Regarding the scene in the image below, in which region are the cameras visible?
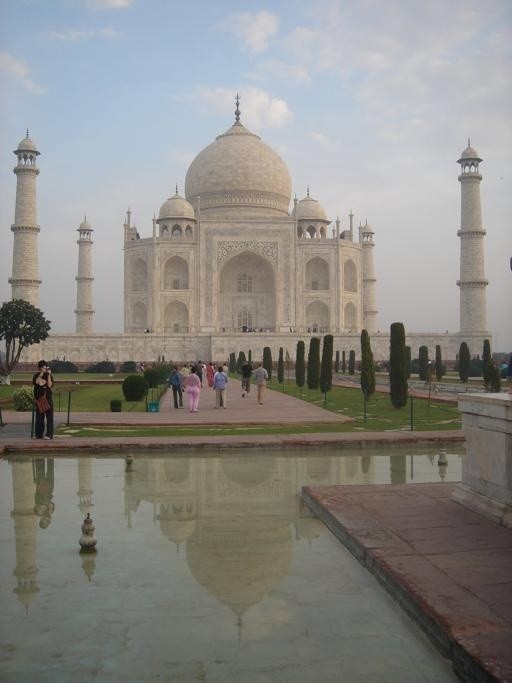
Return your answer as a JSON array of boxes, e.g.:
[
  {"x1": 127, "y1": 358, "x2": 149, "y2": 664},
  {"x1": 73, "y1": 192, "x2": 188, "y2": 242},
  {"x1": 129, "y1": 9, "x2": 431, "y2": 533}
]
[{"x1": 44, "y1": 366, "x2": 50, "y2": 372}]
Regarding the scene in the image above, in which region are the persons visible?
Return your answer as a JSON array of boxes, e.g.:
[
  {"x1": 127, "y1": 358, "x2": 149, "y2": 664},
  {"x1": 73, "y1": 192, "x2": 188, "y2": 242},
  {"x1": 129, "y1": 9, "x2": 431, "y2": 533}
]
[
  {"x1": 240, "y1": 360, "x2": 253, "y2": 397},
  {"x1": 253, "y1": 361, "x2": 269, "y2": 404},
  {"x1": 31, "y1": 360, "x2": 54, "y2": 440},
  {"x1": 501, "y1": 360, "x2": 507, "y2": 369},
  {"x1": 33, "y1": 456, "x2": 56, "y2": 529},
  {"x1": 166, "y1": 359, "x2": 229, "y2": 412}
]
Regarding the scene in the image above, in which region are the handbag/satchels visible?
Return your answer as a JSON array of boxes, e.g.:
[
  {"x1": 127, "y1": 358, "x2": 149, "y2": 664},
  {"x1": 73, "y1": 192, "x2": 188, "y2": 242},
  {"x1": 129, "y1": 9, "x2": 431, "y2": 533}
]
[{"x1": 35, "y1": 397, "x2": 51, "y2": 413}]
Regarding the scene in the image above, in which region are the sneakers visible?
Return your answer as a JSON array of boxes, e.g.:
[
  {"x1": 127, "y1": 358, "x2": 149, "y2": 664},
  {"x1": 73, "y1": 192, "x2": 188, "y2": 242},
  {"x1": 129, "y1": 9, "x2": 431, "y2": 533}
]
[
  {"x1": 43, "y1": 434, "x2": 53, "y2": 440},
  {"x1": 32, "y1": 434, "x2": 42, "y2": 439}
]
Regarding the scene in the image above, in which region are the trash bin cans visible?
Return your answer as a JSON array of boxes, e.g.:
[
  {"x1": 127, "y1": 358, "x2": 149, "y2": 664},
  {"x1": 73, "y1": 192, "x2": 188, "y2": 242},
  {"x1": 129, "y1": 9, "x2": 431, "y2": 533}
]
[
  {"x1": 110, "y1": 400, "x2": 122, "y2": 412},
  {"x1": 149, "y1": 402, "x2": 159, "y2": 412}
]
